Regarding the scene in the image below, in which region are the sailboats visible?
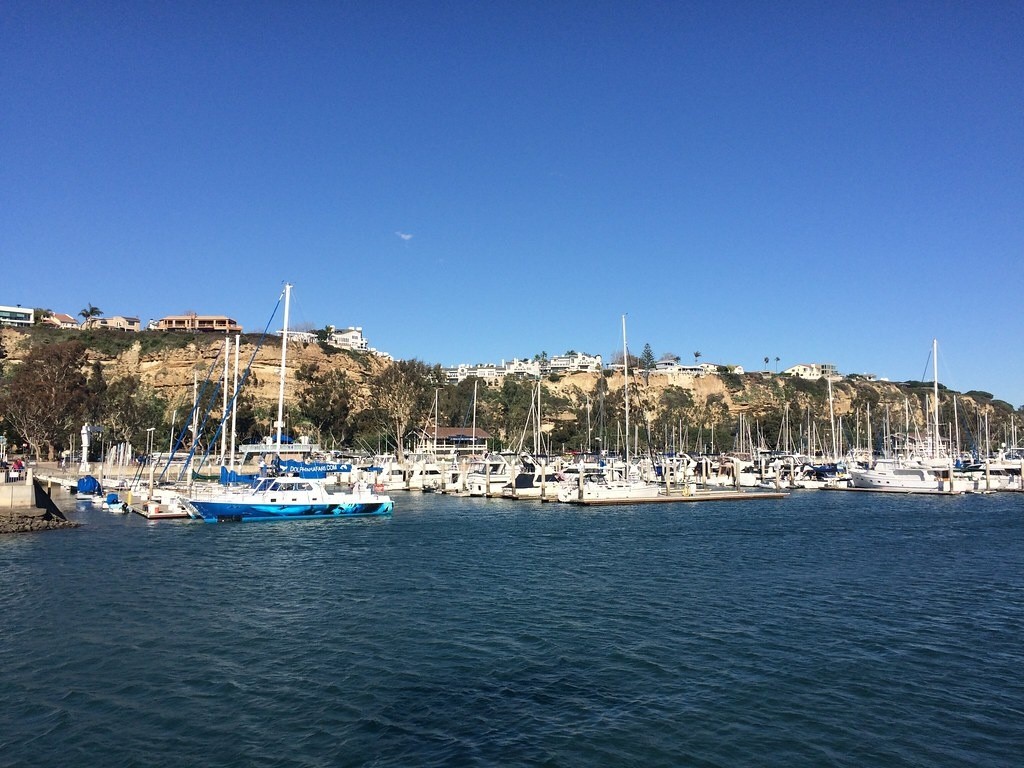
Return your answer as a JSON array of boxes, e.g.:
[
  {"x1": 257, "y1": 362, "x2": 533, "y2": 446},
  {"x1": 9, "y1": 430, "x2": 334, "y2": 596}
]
[{"x1": 77, "y1": 281, "x2": 1024, "y2": 523}]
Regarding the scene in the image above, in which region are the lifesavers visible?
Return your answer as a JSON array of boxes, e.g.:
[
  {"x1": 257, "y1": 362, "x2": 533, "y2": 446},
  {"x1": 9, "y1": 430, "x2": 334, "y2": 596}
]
[
  {"x1": 112, "y1": 499, "x2": 118, "y2": 504},
  {"x1": 122, "y1": 502, "x2": 128, "y2": 508},
  {"x1": 374, "y1": 483, "x2": 384, "y2": 492}
]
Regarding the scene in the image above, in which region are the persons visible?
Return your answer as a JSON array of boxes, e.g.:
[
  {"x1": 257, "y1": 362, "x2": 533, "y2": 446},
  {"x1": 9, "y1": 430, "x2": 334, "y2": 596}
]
[
  {"x1": 12, "y1": 457, "x2": 25, "y2": 472},
  {"x1": 0, "y1": 458, "x2": 8, "y2": 469},
  {"x1": 122, "y1": 502, "x2": 128, "y2": 515}
]
